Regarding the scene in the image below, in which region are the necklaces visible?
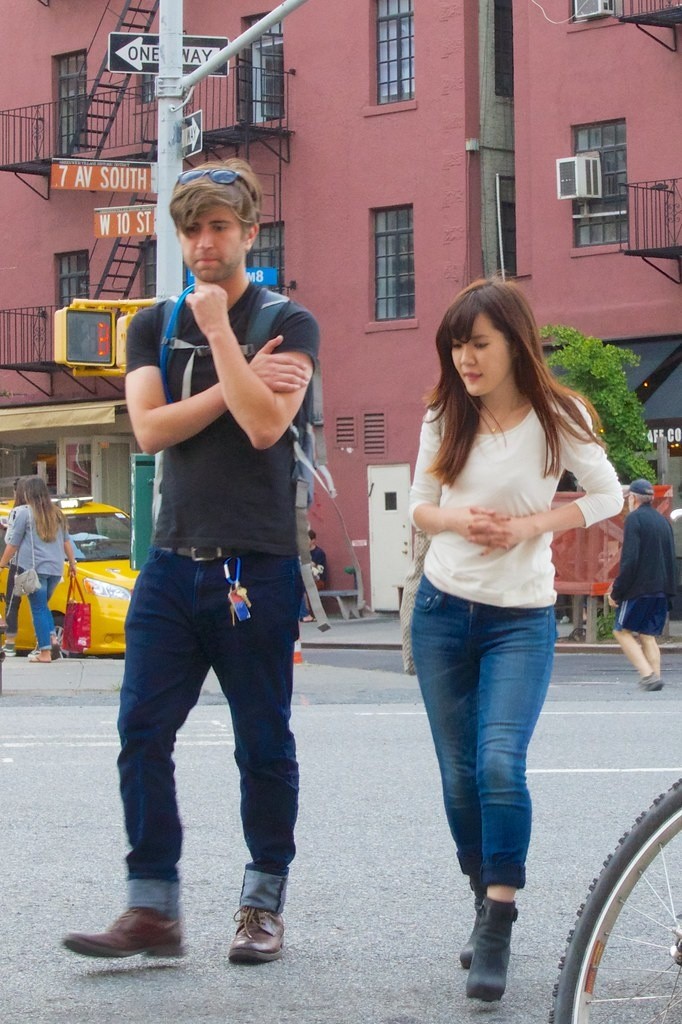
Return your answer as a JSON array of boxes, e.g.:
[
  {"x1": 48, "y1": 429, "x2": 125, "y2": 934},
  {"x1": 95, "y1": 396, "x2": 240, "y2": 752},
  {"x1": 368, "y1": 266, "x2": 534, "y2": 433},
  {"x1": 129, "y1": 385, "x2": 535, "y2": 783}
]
[{"x1": 484, "y1": 410, "x2": 507, "y2": 434}]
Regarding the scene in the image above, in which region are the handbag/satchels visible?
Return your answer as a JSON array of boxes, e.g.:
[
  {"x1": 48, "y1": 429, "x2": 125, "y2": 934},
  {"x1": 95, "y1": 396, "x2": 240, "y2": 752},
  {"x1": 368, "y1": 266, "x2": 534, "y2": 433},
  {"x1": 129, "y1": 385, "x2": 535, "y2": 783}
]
[
  {"x1": 400, "y1": 532, "x2": 432, "y2": 675},
  {"x1": 13, "y1": 569, "x2": 40, "y2": 597},
  {"x1": 62, "y1": 573, "x2": 93, "y2": 652}
]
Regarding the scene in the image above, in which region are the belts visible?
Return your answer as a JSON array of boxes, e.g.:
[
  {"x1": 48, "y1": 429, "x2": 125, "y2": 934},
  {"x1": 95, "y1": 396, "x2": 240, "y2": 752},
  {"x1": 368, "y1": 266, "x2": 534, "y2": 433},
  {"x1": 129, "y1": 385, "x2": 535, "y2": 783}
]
[{"x1": 160, "y1": 545, "x2": 242, "y2": 560}]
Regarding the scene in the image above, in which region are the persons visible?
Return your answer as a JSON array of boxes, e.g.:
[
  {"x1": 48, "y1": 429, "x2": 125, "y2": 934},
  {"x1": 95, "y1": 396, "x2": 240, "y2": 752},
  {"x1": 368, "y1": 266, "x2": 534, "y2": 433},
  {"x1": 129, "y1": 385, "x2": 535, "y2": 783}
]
[
  {"x1": 409, "y1": 283, "x2": 625, "y2": 1003},
  {"x1": 0, "y1": 476, "x2": 78, "y2": 662},
  {"x1": 611, "y1": 479, "x2": 677, "y2": 691},
  {"x1": 0, "y1": 478, "x2": 41, "y2": 657},
  {"x1": 296, "y1": 528, "x2": 327, "y2": 624},
  {"x1": 64, "y1": 158, "x2": 320, "y2": 964}
]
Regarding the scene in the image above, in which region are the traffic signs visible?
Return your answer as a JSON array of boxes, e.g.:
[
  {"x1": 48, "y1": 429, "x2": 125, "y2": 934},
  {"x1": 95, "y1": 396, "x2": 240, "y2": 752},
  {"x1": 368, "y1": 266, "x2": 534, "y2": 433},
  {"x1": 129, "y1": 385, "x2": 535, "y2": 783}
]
[
  {"x1": 106, "y1": 31, "x2": 230, "y2": 78},
  {"x1": 183, "y1": 108, "x2": 203, "y2": 160}
]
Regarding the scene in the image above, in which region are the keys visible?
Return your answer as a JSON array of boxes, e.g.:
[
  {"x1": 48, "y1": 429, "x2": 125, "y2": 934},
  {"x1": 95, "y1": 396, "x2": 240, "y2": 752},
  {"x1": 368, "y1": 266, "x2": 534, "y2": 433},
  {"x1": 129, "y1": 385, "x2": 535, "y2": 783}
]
[{"x1": 230, "y1": 586, "x2": 252, "y2": 627}]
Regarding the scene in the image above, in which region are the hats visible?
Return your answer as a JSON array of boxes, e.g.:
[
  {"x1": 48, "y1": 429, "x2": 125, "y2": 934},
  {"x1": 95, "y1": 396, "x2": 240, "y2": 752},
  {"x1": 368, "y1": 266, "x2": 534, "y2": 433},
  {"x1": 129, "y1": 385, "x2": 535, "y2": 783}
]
[{"x1": 623, "y1": 479, "x2": 654, "y2": 499}]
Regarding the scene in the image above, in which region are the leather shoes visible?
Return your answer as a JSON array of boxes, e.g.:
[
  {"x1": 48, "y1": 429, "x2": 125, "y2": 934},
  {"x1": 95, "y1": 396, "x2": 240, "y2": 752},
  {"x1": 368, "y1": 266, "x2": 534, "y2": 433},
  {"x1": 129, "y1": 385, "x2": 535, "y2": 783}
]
[
  {"x1": 63, "y1": 908, "x2": 182, "y2": 957},
  {"x1": 229, "y1": 907, "x2": 284, "y2": 959}
]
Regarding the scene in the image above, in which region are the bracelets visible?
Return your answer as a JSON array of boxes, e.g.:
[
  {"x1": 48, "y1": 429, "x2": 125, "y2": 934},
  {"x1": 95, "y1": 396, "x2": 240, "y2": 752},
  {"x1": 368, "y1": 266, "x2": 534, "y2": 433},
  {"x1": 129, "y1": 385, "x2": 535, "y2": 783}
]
[{"x1": 69, "y1": 560, "x2": 77, "y2": 566}]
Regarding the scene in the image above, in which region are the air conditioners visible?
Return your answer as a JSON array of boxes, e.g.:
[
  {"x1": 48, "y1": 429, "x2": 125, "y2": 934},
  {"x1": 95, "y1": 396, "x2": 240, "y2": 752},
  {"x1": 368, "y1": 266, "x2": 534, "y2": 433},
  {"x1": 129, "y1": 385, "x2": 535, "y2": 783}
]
[
  {"x1": 557, "y1": 157, "x2": 602, "y2": 200},
  {"x1": 575, "y1": 0, "x2": 615, "y2": 17}
]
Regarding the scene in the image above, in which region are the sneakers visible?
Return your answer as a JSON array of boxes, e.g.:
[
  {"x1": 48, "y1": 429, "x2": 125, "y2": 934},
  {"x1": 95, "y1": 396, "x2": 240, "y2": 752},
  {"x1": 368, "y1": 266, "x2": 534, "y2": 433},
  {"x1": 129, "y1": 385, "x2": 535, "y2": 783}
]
[{"x1": 1, "y1": 642, "x2": 16, "y2": 656}]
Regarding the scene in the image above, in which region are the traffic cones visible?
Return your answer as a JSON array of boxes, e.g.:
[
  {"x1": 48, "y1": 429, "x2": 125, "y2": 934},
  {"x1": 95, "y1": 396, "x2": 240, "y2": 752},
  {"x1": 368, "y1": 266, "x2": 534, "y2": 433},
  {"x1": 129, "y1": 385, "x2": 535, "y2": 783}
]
[{"x1": 293, "y1": 639, "x2": 303, "y2": 665}]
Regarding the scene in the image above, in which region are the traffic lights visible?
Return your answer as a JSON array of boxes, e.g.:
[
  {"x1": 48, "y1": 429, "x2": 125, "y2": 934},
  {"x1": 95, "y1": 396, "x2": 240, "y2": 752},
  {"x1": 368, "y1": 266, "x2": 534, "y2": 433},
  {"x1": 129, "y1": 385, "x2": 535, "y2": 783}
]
[{"x1": 53, "y1": 305, "x2": 116, "y2": 367}]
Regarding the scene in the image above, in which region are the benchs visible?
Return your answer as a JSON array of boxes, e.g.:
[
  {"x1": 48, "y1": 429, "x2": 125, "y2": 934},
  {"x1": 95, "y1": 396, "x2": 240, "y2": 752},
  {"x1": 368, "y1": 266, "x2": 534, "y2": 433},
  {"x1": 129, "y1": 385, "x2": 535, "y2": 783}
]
[{"x1": 318, "y1": 589, "x2": 363, "y2": 621}]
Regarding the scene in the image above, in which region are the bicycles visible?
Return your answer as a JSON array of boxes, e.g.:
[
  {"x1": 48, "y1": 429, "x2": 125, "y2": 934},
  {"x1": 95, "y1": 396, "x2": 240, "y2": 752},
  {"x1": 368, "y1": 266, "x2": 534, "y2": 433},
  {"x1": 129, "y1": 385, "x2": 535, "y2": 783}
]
[{"x1": 548, "y1": 776, "x2": 681, "y2": 1024}]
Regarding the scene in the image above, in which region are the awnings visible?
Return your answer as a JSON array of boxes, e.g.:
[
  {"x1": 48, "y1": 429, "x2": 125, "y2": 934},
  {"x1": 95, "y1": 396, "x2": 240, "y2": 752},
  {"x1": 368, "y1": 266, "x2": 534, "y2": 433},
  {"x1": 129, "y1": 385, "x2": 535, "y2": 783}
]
[{"x1": 534, "y1": 335, "x2": 682, "y2": 457}]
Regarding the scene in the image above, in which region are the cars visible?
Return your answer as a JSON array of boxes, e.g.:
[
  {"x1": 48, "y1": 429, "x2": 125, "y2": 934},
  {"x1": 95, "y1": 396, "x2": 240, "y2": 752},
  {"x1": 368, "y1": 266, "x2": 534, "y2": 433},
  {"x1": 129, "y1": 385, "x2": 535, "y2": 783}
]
[{"x1": 0, "y1": 497, "x2": 142, "y2": 658}]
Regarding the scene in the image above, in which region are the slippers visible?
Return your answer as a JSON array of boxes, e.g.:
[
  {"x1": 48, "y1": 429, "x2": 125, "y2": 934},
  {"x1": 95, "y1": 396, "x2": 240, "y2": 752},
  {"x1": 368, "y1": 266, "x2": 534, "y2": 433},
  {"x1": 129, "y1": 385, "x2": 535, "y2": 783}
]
[
  {"x1": 50, "y1": 643, "x2": 60, "y2": 660},
  {"x1": 29, "y1": 656, "x2": 49, "y2": 663}
]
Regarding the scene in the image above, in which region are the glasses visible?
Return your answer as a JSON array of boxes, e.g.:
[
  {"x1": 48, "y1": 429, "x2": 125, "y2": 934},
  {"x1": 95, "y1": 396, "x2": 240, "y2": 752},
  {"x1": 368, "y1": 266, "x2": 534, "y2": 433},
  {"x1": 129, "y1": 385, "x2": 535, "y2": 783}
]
[{"x1": 172, "y1": 168, "x2": 258, "y2": 222}]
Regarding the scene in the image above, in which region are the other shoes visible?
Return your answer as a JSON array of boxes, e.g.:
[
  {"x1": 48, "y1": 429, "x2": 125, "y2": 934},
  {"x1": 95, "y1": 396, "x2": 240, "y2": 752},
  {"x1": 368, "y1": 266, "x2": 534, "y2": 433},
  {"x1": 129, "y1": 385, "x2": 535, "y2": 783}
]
[
  {"x1": 300, "y1": 616, "x2": 316, "y2": 621},
  {"x1": 639, "y1": 672, "x2": 665, "y2": 692}
]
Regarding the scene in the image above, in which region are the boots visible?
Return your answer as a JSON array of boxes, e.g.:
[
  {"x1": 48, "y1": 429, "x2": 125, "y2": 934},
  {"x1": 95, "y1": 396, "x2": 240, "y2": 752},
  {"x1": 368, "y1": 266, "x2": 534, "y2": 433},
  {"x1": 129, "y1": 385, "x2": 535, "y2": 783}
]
[
  {"x1": 459, "y1": 888, "x2": 489, "y2": 968},
  {"x1": 467, "y1": 896, "x2": 518, "y2": 1001}
]
